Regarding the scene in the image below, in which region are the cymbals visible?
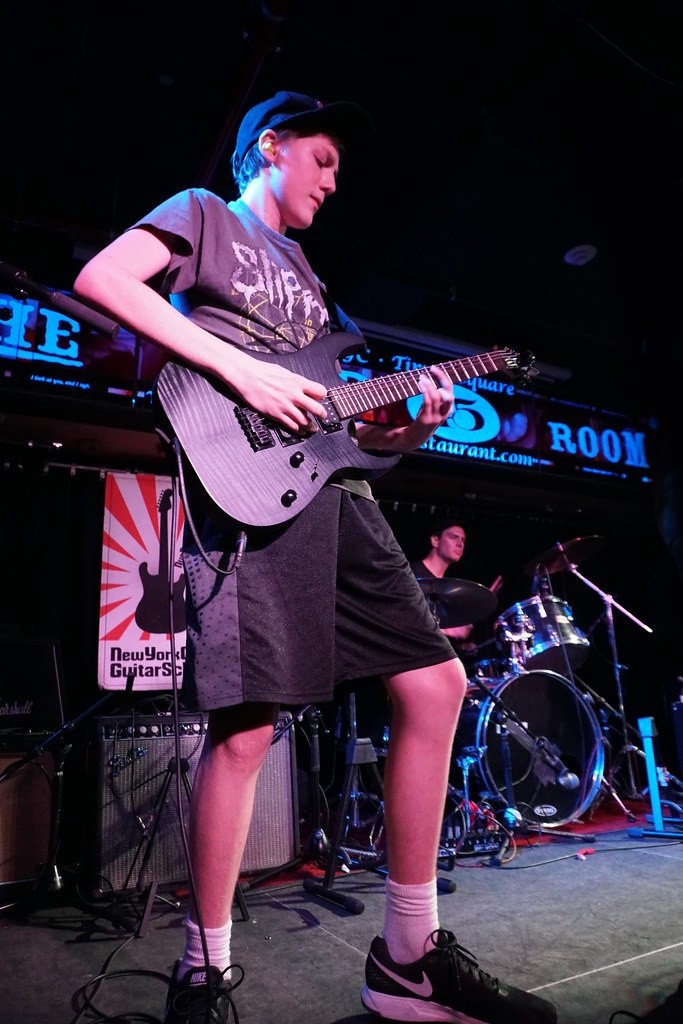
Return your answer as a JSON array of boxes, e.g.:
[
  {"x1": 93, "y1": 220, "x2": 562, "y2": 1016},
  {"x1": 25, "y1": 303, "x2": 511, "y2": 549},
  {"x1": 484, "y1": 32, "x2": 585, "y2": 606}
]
[
  {"x1": 528, "y1": 534, "x2": 605, "y2": 576},
  {"x1": 417, "y1": 576, "x2": 500, "y2": 629}
]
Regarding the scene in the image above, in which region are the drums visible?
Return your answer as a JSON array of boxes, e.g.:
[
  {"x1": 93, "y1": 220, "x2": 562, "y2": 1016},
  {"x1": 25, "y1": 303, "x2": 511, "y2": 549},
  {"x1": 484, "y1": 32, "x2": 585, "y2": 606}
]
[
  {"x1": 495, "y1": 594, "x2": 590, "y2": 675},
  {"x1": 463, "y1": 658, "x2": 510, "y2": 681},
  {"x1": 451, "y1": 670, "x2": 605, "y2": 828}
]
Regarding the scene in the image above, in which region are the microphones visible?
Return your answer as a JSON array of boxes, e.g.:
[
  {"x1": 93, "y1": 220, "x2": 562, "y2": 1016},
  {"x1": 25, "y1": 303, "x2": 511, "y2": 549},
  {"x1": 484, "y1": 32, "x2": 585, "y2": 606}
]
[
  {"x1": 556, "y1": 773, "x2": 580, "y2": 789},
  {"x1": 530, "y1": 564, "x2": 542, "y2": 595}
]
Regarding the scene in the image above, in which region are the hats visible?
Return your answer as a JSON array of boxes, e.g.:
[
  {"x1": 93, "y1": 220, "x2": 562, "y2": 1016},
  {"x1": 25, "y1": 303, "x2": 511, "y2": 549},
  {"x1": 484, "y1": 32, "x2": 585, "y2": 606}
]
[{"x1": 231, "y1": 91, "x2": 377, "y2": 168}]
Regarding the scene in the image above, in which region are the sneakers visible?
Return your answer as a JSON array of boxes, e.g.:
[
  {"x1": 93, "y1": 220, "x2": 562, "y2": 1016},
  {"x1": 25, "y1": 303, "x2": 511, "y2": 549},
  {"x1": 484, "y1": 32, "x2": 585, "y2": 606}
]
[
  {"x1": 162, "y1": 958, "x2": 244, "y2": 1024},
  {"x1": 360, "y1": 927, "x2": 558, "y2": 1024}
]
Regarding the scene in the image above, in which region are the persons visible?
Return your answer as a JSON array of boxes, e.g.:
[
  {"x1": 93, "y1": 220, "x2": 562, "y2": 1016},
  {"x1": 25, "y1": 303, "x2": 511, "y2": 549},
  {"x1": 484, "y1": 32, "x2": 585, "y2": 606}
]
[
  {"x1": 73, "y1": 91, "x2": 558, "y2": 1024},
  {"x1": 407, "y1": 519, "x2": 474, "y2": 648}
]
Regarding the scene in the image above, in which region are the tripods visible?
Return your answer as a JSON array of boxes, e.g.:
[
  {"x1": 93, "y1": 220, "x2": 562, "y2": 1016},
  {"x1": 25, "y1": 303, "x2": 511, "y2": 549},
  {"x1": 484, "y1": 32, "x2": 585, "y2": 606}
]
[
  {"x1": 240, "y1": 690, "x2": 456, "y2": 894},
  {"x1": 0, "y1": 690, "x2": 144, "y2": 936},
  {"x1": 466, "y1": 668, "x2": 595, "y2": 867},
  {"x1": 559, "y1": 552, "x2": 683, "y2": 819}
]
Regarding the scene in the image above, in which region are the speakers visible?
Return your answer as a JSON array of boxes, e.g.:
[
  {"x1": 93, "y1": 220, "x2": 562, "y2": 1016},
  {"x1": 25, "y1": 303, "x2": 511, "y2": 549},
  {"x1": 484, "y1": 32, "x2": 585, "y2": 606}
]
[
  {"x1": 0, "y1": 731, "x2": 61, "y2": 885},
  {"x1": 87, "y1": 709, "x2": 301, "y2": 902}
]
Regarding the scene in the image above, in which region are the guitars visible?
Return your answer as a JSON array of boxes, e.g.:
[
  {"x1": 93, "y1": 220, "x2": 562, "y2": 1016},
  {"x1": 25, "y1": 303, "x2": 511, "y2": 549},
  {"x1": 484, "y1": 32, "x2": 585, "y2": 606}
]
[{"x1": 155, "y1": 331, "x2": 541, "y2": 527}]
